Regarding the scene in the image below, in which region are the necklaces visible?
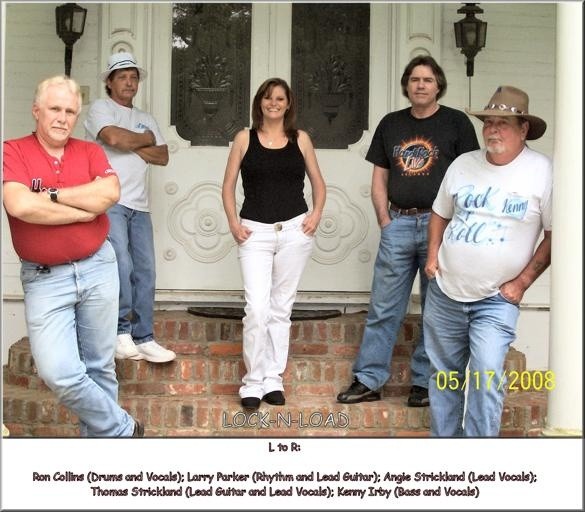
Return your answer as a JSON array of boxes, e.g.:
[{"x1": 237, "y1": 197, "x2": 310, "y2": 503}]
[{"x1": 260, "y1": 131, "x2": 285, "y2": 148}]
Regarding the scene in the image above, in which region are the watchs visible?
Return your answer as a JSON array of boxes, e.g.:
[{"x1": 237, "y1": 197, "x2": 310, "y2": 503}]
[{"x1": 47, "y1": 185, "x2": 57, "y2": 205}]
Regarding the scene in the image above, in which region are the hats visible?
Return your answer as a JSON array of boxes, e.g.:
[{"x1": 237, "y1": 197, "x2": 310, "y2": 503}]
[
  {"x1": 466, "y1": 86, "x2": 546, "y2": 140},
  {"x1": 99, "y1": 53, "x2": 148, "y2": 83}
]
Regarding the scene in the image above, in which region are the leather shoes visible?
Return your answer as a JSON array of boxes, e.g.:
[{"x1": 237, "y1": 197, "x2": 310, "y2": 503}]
[
  {"x1": 263, "y1": 391, "x2": 285, "y2": 405},
  {"x1": 131, "y1": 419, "x2": 144, "y2": 437},
  {"x1": 241, "y1": 397, "x2": 260, "y2": 409}
]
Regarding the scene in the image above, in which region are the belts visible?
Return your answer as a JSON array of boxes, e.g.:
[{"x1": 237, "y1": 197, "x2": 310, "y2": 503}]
[{"x1": 390, "y1": 203, "x2": 432, "y2": 216}]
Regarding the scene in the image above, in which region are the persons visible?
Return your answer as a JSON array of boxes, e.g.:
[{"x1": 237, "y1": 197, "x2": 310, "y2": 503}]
[
  {"x1": 1, "y1": 76, "x2": 146, "y2": 437},
  {"x1": 338, "y1": 55, "x2": 483, "y2": 408},
  {"x1": 221, "y1": 78, "x2": 327, "y2": 409},
  {"x1": 83, "y1": 52, "x2": 178, "y2": 365},
  {"x1": 422, "y1": 87, "x2": 553, "y2": 437}
]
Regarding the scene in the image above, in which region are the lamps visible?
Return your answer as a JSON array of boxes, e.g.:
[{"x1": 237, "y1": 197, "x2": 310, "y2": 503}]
[
  {"x1": 452, "y1": 1, "x2": 489, "y2": 113},
  {"x1": 52, "y1": 1, "x2": 89, "y2": 79}
]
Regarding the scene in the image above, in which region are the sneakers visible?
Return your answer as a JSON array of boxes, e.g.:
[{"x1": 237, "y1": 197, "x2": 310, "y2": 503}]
[
  {"x1": 115, "y1": 334, "x2": 139, "y2": 361},
  {"x1": 408, "y1": 386, "x2": 429, "y2": 406},
  {"x1": 338, "y1": 381, "x2": 380, "y2": 403},
  {"x1": 135, "y1": 341, "x2": 176, "y2": 363}
]
